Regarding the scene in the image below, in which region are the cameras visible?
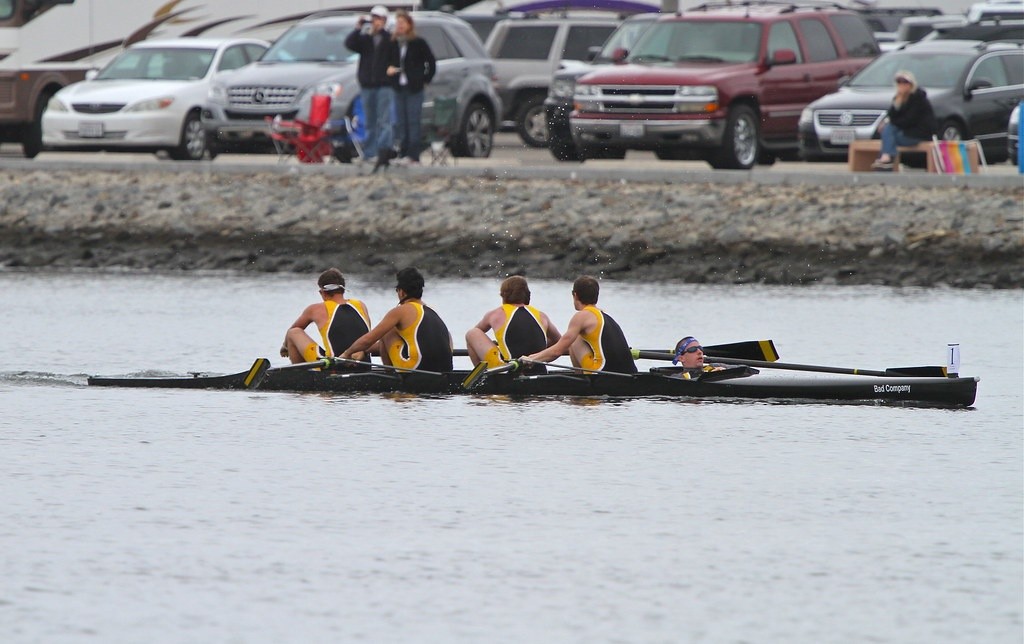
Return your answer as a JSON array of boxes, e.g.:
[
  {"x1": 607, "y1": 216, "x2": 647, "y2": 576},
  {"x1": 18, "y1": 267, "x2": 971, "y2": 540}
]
[{"x1": 361, "y1": 19, "x2": 373, "y2": 24}]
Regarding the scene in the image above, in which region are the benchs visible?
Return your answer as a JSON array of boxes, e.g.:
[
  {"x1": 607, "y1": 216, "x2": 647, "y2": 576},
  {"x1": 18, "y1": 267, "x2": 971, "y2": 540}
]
[{"x1": 849, "y1": 141, "x2": 980, "y2": 176}]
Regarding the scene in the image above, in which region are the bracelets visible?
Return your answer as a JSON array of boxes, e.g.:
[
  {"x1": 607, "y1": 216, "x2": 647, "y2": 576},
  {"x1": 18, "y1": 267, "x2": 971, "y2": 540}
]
[{"x1": 283, "y1": 343, "x2": 288, "y2": 350}]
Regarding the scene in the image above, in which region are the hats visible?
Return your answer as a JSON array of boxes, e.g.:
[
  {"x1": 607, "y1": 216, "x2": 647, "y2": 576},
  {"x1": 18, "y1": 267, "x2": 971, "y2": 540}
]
[
  {"x1": 894, "y1": 70, "x2": 915, "y2": 83},
  {"x1": 371, "y1": 5, "x2": 390, "y2": 17}
]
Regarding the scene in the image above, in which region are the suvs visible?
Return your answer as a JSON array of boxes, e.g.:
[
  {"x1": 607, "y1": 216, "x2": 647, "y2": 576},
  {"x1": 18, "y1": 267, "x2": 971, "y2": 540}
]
[
  {"x1": 199, "y1": 9, "x2": 507, "y2": 164},
  {"x1": 567, "y1": 1, "x2": 885, "y2": 171}
]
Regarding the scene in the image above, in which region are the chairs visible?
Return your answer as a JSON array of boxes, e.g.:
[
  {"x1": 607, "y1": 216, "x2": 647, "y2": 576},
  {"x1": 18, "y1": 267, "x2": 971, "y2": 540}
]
[
  {"x1": 330, "y1": 93, "x2": 397, "y2": 167},
  {"x1": 266, "y1": 94, "x2": 333, "y2": 165},
  {"x1": 420, "y1": 97, "x2": 461, "y2": 167}
]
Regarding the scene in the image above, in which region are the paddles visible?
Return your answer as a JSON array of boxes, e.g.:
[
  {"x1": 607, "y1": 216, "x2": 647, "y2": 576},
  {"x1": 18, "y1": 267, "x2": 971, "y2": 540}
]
[
  {"x1": 629, "y1": 349, "x2": 949, "y2": 376},
  {"x1": 460, "y1": 358, "x2": 529, "y2": 392},
  {"x1": 243, "y1": 355, "x2": 352, "y2": 390},
  {"x1": 450, "y1": 339, "x2": 779, "y2": 362}
]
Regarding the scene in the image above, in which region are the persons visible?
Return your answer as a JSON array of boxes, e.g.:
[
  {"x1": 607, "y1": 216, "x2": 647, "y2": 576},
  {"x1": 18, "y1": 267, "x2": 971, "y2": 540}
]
[
  {"x1": 518, "y1": 275, "x2": 638, "y2": 374},
  {"x1": 343, "y1": 5, "x2": 400, "y2": 161},
  {"x1": 378, "y1": 12, "x2": 436, "y2": 167},
  {"x1": 279, "y1": 268, "x2": 371, "y2": 374},
  {"x1": 871, "y1": 68, "x2": 936, "y2": 171},
  {"x1": 669, "y1": 336, "x2": 727, "y2": 379},
  {"x1": 465, "y1": 275, "x2": 562, "y2": 376},
  {"x1": 338, "y1": 267, "x2": 453, "y2": 373}
]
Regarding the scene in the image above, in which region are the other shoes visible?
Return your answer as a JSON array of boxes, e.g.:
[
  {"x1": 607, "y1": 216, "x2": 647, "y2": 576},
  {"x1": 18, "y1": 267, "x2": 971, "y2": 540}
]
[{"x1": 872, "y1": 160, "x2": 893, "y2": 170}]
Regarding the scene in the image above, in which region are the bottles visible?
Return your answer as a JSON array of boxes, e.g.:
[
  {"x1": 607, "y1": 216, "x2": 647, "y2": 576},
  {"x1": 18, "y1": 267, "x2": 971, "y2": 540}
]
[{"x1": 271, "y1": 113, "x2": 282, "y2": 128}]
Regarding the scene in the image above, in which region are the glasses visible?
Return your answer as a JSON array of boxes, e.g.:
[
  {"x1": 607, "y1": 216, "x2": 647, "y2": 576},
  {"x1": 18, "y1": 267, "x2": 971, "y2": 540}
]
[{"x1": 682, "y1": 346, "x2": 703, "y2": 355}]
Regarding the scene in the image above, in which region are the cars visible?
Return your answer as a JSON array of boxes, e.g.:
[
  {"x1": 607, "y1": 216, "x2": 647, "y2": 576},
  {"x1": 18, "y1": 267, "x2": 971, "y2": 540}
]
[
  {"x1": 453, "y1": 1, "x2": 683, "y2": 151},
  {"x1": 797, "y1": 0, "x2": 1023, "y2": 170},
  {"x1": 40, "y1": 36, "x2": 295, "y2": 161}
]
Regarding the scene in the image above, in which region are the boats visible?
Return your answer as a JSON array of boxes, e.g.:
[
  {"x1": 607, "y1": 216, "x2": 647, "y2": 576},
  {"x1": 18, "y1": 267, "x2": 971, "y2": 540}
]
[{"x1": 86, "y1": 364, "x2": 980, "y2": 413}]
